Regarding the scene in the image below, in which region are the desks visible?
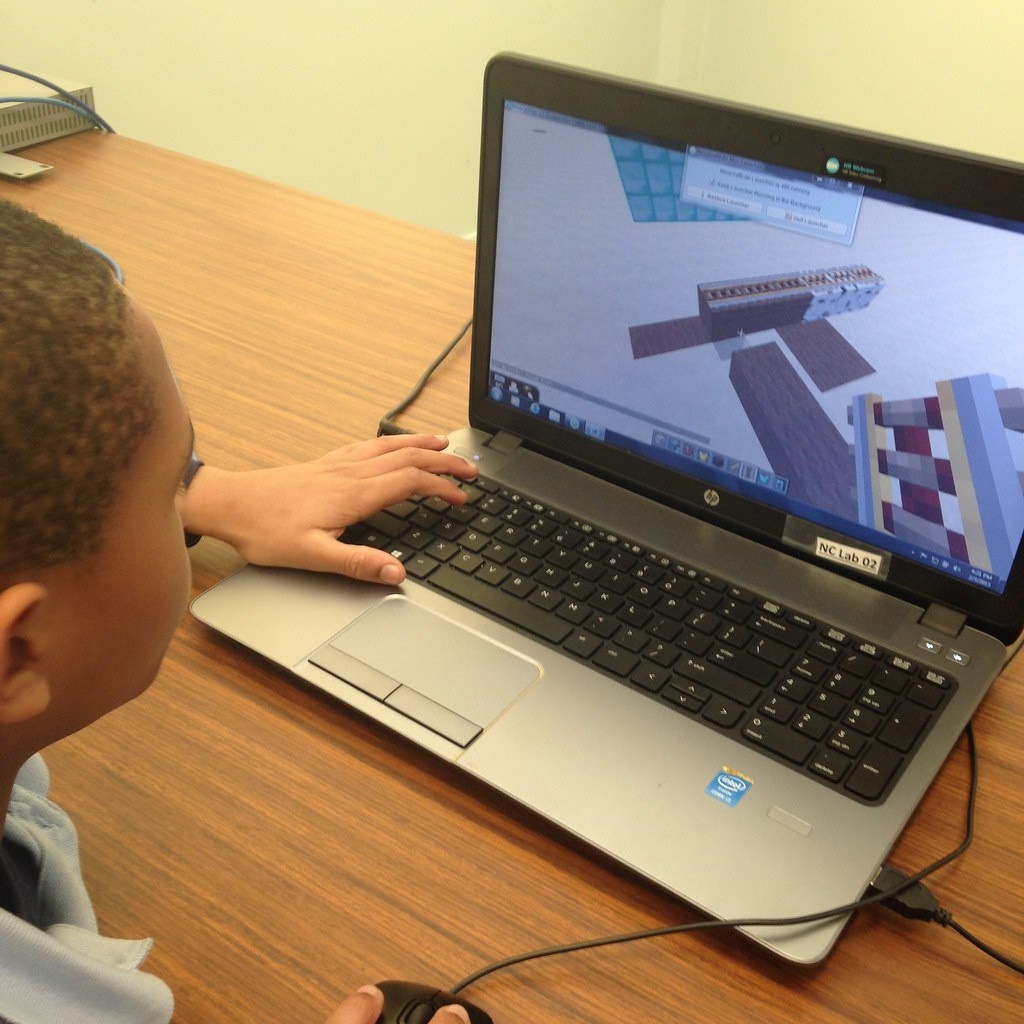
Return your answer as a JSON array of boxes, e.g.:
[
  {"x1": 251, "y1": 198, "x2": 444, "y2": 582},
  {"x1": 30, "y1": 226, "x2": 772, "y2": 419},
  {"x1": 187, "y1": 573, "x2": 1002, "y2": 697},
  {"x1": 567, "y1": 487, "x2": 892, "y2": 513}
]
[{"x1": 0, "y1": 129, "x2": 1024, "y2": 1024}]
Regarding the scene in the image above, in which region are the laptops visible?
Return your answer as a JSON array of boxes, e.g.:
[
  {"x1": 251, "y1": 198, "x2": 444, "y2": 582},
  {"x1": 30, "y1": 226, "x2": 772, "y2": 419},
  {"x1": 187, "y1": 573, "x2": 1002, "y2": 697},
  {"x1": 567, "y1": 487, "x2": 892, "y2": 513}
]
[{"x1": 188, "y1": 52, "x2": 1024, "y2": 971}]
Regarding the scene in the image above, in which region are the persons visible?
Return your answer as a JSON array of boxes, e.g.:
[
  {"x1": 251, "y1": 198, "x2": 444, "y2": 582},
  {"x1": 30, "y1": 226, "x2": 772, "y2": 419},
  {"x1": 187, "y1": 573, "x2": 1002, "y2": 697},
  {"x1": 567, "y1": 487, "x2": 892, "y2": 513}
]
[{"x1": 2, "y1": 200, "x2": 479, "y2": 1022}]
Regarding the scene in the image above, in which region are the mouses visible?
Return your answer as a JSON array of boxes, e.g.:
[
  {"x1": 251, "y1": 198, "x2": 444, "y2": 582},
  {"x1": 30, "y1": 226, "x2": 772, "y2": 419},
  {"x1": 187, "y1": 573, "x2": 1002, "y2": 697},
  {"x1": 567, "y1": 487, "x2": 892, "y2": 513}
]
[{"x1": 371, "y1": 980, "x2": 493, "y2": 1024}]
